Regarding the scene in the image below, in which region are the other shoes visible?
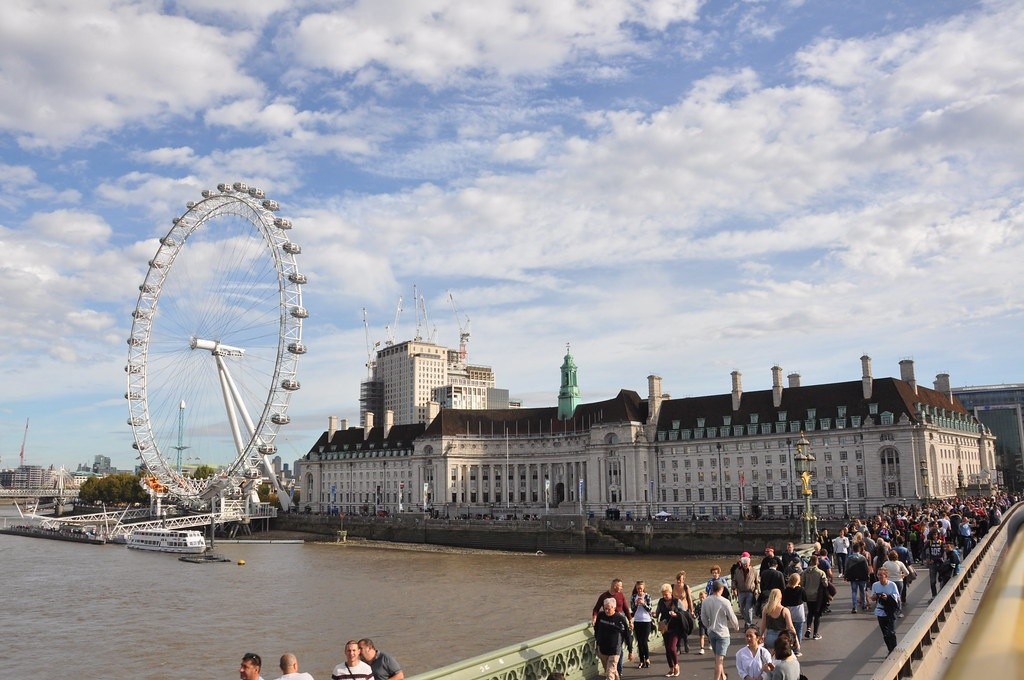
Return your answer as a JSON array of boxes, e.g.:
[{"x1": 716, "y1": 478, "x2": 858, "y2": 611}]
[
  {"x1": 709, "y1": 644, "x2": 712, "y2": 650},
  {"x1": 674, "y1": 665, "x2": 680, "y2": 676},
  {"x1": 646, "y1": 660, "x2": 650, "y2": 668},
  {"x1": 685, "y1": 644, "x2": 689, "y2": 652},
  {"x1": 795, "y1": 652, "x2": 802, "y2": 657},
  {"x1": 838, "y1": 573, "x2": 842, "y2": 579},
  {"x1": 676, "y1": 648, "x2": 681, "y2": 655},
  {"x1": 813, "y1": 633, "x2": 822, "y2": 640},
  {"x1": 638, "y1": 663, "x2": 644, "y2": 668},
  {"x1": 863, "y1": 606, "x2": 868, "y2": 610},
  {"x1": 852, "y1": 608, "x2": 857, "y2": 613},
  {"x1": 824, "y1": 609, "x2": 831, "y2": 613},
  {"x1": 804, "y1": 630, "x2": 810, "y2": 638},
  {"x1": 699, "y1": 648, "x2": 704, "y2": 655},
  {"x1": 665, "y1": 670, "x2": 675, "y2": 677},
  {"x1": 897, "y1": 613, "x2": 905, "y2": 618}
]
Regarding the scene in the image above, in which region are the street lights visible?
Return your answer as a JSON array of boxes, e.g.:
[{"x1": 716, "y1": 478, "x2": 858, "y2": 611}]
[
  {"x1": 920, "y1": 457, "x2": 930, "y2": 507},
  {"x1": 791, "y1": 430, "x2": 820, "y2": 544},
  {"x1": 956, "y1": 465, "x2": 969, "y2": 503}
]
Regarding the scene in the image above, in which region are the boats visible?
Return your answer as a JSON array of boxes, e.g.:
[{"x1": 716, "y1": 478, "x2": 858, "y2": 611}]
[{"x1": 126, "y1": 529, "x2": 207, "y2": 555}]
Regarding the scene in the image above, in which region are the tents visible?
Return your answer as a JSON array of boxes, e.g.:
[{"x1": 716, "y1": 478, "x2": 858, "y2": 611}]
[{"x1": 655, "y1": 511, "x2": 671, "y2": 518}]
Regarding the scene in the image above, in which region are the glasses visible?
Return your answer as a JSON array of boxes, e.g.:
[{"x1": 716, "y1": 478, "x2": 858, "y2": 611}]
[{"x1": 245, "y1": 653, "x2": 258, "y2": 665}]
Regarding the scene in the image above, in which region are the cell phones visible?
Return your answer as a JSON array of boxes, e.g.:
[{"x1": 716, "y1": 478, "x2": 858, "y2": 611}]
[{"x1": 638, "y1": 598, "x2": 642, "y2": 602}]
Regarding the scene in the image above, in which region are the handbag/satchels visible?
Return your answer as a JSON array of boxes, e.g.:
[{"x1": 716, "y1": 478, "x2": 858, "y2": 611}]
[{"x1": 658, "y1": 619, "x2": 668, "y2": 633}]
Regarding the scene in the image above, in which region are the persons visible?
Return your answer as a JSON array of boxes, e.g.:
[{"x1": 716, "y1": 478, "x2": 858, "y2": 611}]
[
  {"x1": 239, "y1": 638, "x2": 404, "y2": 680},
  {"x1": 591, "y1": 490, "x2": 1024, "y2": 680}
]
